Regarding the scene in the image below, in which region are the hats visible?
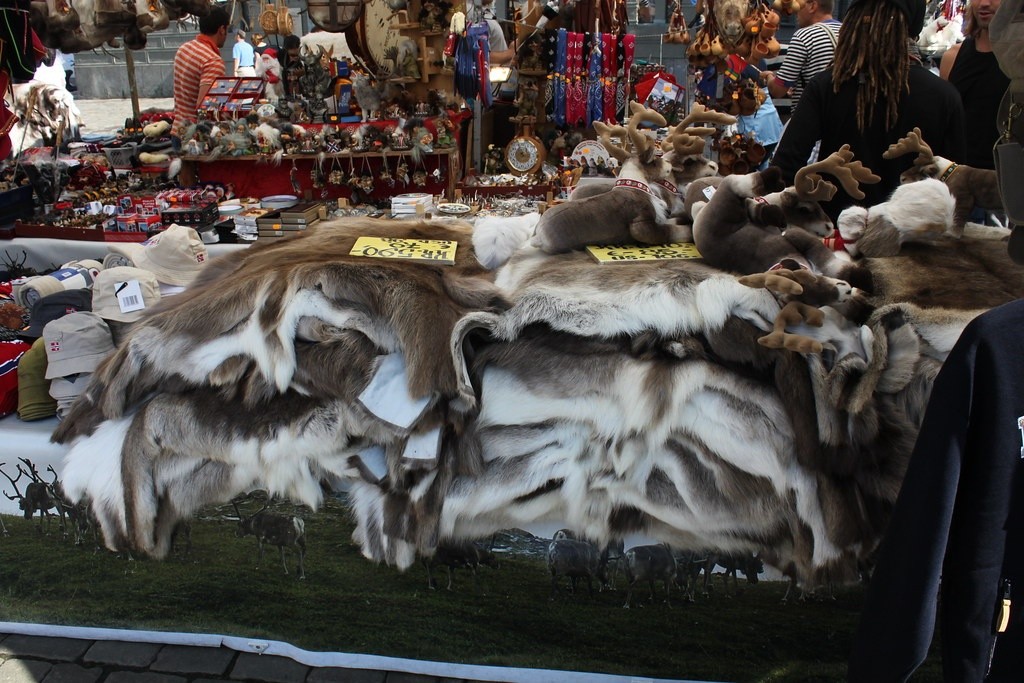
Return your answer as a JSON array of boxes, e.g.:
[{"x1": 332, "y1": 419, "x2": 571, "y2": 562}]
[{"x1": 0, "y1": 224, "x2": 210, "y2": 420}]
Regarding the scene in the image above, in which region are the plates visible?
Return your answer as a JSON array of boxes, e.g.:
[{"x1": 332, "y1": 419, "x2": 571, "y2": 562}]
[
  {"x1": 436, "y1": 203, "x2": 471, "y2": 214},
  {"x1": 572, "y1": 141, "x2": 610, "y2": 167}
]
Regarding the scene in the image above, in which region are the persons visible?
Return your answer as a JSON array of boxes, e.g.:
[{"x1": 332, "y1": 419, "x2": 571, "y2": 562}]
[
  {"x1": 694, "y1": 0, "x2": 1023, "y2": 269},
  {"x1": 170, "y1": 8, "x2": 231, "y2": 147},
  {"x1": 233, "y1": 29, "x2": 303, "y2": 100},
  {"x1": 485, "y1": 13, "x2": 521, "y2": 67}
]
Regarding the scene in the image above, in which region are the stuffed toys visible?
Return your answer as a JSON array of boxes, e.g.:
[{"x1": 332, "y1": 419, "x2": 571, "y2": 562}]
[{"x1": 527, "y1": 100, "x2": 1004, "y2": 355}]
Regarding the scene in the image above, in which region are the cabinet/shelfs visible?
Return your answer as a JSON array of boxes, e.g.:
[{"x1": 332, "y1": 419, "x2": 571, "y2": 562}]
[{"x1": 180, "y1": 120, "x2": 460, "y2": 203}]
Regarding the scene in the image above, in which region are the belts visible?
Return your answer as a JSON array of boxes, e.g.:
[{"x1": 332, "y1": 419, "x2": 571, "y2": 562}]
[{"x1": 239, "y1": 66, "x2": 253, "y2": 67}]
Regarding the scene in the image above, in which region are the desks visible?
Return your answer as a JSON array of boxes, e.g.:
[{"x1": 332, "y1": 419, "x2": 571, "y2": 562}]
[{"x1": 0, "y1": 238, "x2": 253, "y2": 272}]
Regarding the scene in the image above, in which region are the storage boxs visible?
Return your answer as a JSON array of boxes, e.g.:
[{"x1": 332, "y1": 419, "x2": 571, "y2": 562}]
[
  {"x1": 15, "y1": 193, "x2": 328, "y2": 242},
  {"x1": 390, "y1": 193, "x2": 433, "y2": 220},
  {"x1": 196, "y1": 76, "x2": 266, "y2": 120}
]
